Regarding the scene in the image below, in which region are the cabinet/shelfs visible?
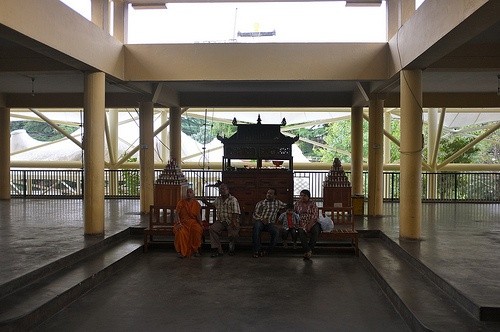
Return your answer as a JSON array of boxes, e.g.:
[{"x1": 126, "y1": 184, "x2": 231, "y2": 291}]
[{"x1": 216, "y1": 114, "x2": 299, "y2": 225}]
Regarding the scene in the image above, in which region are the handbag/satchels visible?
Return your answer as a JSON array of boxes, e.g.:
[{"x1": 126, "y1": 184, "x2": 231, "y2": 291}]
[{"x1": 320, "y1": 213, "x2": 334, "y2": 233}]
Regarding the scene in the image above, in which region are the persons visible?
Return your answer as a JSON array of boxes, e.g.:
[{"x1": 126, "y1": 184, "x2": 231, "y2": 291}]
[
  {"x1": 201, "y1": 184, "x2": 241, "y2": 257},
  {"x1": 173, "y1": 188, "x2": 204, "y2": 257},
  {"x1": 252, "y1": 188, "x2": 287, "y2": 257},
  {"x1": 277, "y1": 204, "x2": 300, "y2": 250},
  {"x1": 294, "y1": 189, "x2": 321, "y2": 259}
]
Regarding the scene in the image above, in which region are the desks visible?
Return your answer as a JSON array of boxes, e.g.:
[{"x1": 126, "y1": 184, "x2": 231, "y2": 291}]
[
  {"x1": 323, "y1": 186, "x2": 352, "y2": 217},
  {"x1": 154, "y1": 184, "x2": 188, "y2": 216}
]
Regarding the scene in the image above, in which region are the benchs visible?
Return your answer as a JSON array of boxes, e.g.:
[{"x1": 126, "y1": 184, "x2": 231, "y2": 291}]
[{"x1": 144, "y1": 205, "x2": 359, "y2": 257}]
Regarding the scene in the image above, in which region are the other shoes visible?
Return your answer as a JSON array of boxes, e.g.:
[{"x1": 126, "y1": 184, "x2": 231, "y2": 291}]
[
  {"x1": 229, "y1": 250, "x2": 235, "y2": 256},
  {"x1": 261, "y1": 249, "x2": 269, "y2": 257},
  {"x1": 304, "y1": 252, "x2": 312, "y2": 261},
  {"x1": 293, "y1": 243, "x2": 296, "y2": 249},
  {"x1": 217, "y1": 250, "x2": 223, "y2": 256},
  {"x1": 194, "y1": 251, "x2": 200, "y2": 257},
  {"x1": 177, "y1": 252, "x2": 185, "y2": 257},
  {"x1": 283, "y1": 244, "x2": 288, "y2": 249},
  {"x1": 253, "y1": 252, "x2": 260, "y2": 258}
]
